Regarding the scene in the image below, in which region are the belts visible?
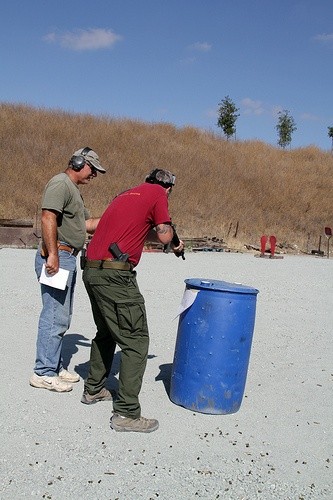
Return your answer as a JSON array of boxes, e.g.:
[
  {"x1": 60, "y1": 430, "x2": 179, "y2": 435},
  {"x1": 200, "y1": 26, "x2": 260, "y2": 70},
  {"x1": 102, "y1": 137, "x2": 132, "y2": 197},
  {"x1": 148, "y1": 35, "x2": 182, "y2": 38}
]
[
  {"x1": 56, "y1": 243, "x2": 73, "y2": 253},
  {"x1": 85, "y1": 260, "x2": 130, "y2": 271}
]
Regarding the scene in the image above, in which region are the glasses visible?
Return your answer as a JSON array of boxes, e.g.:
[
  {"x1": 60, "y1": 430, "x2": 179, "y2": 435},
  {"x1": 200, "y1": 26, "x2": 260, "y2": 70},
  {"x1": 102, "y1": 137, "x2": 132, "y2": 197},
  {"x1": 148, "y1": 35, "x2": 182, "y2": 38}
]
[{"x1": 86, "y1": 162, "x2": 97, "y2": 174}]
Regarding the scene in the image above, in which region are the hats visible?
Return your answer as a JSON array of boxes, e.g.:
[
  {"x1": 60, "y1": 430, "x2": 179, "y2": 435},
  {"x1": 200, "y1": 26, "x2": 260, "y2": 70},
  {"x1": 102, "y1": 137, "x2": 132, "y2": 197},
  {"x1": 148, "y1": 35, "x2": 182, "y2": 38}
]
[
  {"x1": 145, "y1": 167, "x2": 176, "y2": 188},
  {"x1": 73, "y1": 147, "x2": 106, "y2": 175}
]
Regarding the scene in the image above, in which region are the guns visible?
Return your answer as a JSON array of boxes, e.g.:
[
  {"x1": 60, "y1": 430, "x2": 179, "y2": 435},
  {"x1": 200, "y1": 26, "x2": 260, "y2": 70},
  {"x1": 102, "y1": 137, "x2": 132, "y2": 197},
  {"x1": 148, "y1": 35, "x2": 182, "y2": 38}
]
[
  {"x1": 150, "y1": 223, "x2": 186, "y2": 261},
  {"x1": 108, "y1": 242, "x2": 130, "y2": 263}
]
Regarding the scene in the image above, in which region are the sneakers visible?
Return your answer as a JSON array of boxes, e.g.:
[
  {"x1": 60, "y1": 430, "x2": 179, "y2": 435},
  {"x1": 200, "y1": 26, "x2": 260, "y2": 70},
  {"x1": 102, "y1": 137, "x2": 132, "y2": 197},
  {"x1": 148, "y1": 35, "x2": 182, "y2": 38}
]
[
  {"x1": 109, "y1": 410, "x2": 160, "y2": 433},
  {"x1": 57, "y1": 369, "x2": 81, "y2": 383},
  {"x1": 29, "y1": 372, "x2": 73, "y2": 392},
  {"x1": 80, "y1": 388, "x2": 116, "y2": 405}
]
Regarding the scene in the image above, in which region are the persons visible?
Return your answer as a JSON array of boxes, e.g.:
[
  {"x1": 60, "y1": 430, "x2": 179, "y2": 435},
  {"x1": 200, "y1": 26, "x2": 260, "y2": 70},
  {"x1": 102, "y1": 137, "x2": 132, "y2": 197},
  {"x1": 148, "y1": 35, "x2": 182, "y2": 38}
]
[
  {"x1": 81, "y1": 168, "x2": 185, "y2": 433},
  {"x1": 29, "y1": 147, "x2": 108, "y2": 392}
]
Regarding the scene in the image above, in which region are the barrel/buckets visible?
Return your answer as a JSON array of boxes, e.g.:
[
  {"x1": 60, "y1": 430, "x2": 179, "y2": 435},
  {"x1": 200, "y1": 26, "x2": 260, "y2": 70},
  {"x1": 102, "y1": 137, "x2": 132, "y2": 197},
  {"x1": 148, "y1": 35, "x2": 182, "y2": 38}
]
[{"x1": 170, "y1": 278, "x2": 259, "y2": 415}]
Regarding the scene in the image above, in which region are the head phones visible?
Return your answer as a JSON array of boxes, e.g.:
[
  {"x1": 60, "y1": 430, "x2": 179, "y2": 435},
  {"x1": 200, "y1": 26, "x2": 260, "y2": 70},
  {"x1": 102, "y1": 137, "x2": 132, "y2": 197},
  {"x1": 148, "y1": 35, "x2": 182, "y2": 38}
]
[{"x1": 71, "y1": 146, "x2": 93, "y2": 170}]
